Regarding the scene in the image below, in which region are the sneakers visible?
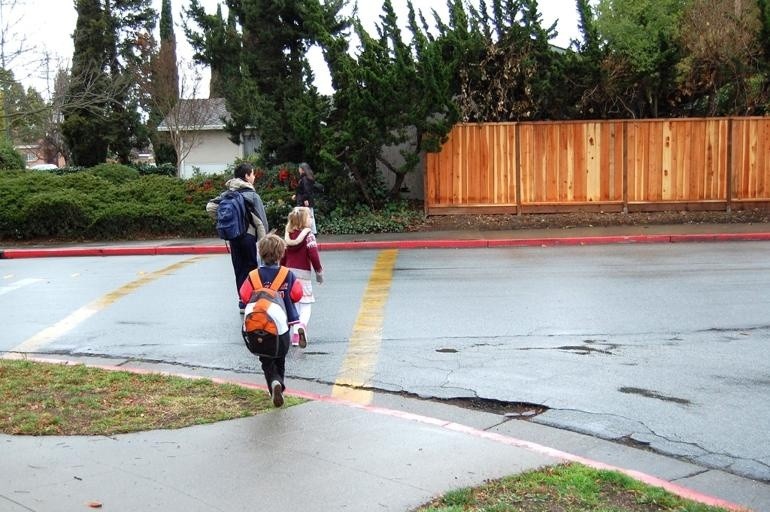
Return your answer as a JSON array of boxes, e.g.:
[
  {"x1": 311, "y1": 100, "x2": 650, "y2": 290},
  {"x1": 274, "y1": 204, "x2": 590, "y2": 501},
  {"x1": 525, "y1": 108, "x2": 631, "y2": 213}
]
[
  {"x1": 272, "y1": 380, "x2": 284, "y2": 407},
  {"x1": 238, "y1": 301, "x2": 245, "y2": 314},
  {"x1": 292, "y1": 327, "x2": 307, "y2": 348}
]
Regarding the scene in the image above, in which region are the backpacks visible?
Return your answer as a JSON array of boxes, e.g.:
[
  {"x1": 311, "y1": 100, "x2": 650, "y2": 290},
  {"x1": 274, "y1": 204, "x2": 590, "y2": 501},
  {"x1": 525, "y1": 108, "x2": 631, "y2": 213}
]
[
  {"x1": 216, "y1": 187, "x2": 254, "y2": 240},
  {"x1": 241, "y1": 266, "x2": 289, "y2": 359}
]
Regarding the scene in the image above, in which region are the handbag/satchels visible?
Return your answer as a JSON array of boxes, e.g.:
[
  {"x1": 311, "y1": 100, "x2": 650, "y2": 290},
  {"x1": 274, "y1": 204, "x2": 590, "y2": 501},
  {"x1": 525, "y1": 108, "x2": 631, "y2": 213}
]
[{"x1": 295, "y1": 206, "x2": 317, "y2": 234}]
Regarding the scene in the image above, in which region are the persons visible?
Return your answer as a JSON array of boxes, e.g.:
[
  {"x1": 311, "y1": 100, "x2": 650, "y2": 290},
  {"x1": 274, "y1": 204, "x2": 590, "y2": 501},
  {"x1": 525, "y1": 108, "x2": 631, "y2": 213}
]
[
  {"x1": 206, "y1": 164, "x2": 270, "y2": 315},
  {"x1": 239, "y1": 233, "x2": 304, "y2": 407},
  {"x1": 290, "y1": 162, "x2": 318, "y2": 236},
  {"x1": 277, "y1": 207, "x2": 325, "y2": 348}
]
